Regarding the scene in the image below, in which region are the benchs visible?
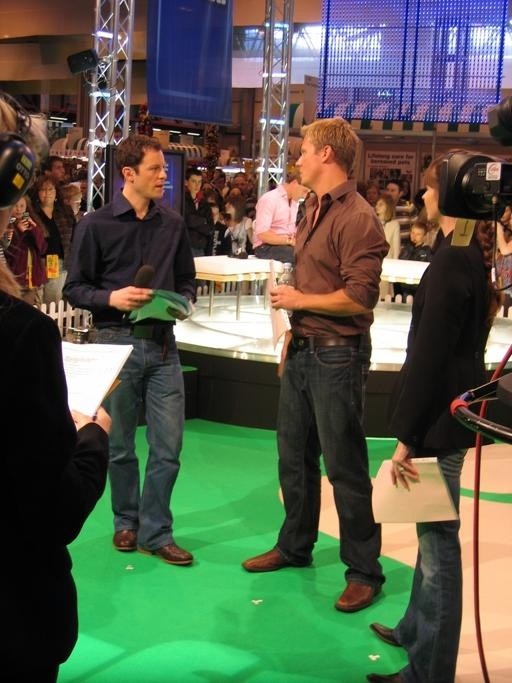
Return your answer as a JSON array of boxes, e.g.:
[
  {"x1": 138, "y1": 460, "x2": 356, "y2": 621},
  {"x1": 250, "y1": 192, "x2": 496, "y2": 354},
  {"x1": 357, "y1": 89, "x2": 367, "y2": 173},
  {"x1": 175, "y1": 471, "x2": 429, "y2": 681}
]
[{"x1": 193, "y1": 253, "x2": 291, "y2": 321}]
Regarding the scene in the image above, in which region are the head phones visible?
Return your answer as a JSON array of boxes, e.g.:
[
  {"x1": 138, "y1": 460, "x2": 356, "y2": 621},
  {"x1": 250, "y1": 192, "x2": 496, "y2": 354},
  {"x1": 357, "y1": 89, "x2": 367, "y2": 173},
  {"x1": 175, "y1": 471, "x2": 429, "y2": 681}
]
[{"x1": 0, "y1": 88, "x2": 34, "y2": 209}]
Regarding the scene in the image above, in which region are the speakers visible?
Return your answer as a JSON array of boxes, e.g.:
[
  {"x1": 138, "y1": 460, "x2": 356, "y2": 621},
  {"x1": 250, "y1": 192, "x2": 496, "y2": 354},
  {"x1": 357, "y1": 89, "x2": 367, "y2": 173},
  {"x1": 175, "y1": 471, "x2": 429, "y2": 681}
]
[{"x1": 66, "y1": 47, "x2": 100, "y2": 75}]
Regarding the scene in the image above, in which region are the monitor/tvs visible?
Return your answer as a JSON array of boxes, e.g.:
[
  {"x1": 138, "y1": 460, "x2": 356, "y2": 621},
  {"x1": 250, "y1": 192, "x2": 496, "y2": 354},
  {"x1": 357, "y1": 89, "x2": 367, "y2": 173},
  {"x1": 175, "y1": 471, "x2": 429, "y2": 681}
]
[{"x1": 106, "y1": 142, "x2": 188, "y2": 218}]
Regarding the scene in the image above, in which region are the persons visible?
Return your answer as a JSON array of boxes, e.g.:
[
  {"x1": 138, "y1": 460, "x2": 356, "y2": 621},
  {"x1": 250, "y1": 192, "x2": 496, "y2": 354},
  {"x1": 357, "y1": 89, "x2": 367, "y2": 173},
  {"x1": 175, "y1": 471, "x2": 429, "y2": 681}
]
[
  {"x1": 240, "y1": 115, "x2": 391, "y2": 614},
  {"x1": 395, "y1": 222, "x2": 432, "y2": 304},
  {"x1": 492, "y1": 201, "x2": 511, "y2": 303},
  {"x1": 41, "y1": 155, "x2": 65, "y2": 186},
  {"x1": 181, "y1": 169, "x2": 214, "y2": 257},
  {"x1": 31, "y1": 177, "x2": 78, "y2": 267},
  {"x1": 379, "y1": 180, "x2": 420, "y2": 258},
  {"x1": 4, "y1": 194, "x2": 50, "y2": 293},
  {"x1": 204, "y1": 163, "x2": 254, "y2": 205},
  {"x1": 204, "y1": 203, "x2": 232, "y2": 255},
  {"x1": 252, "y1": 165, "x2": 311, "y2": 263},
  {"x1": 1, "y1": 97, "x2": 113, "y2": 683},
  {"x1": 364, "y1": 151, "x2": 494, "y2": 682},
  {"x1": 58, "y1": 184, "x2": 82, "y2": 223},
  {"x1": 222, "y1": 200, "x2": 252, "y2": 253},
  {"x1": 62, "y1": 134, "x2": 199, "y2": 568},
  {"x1": 369, "y1": 194, "x2": 400, "y2": 299}
]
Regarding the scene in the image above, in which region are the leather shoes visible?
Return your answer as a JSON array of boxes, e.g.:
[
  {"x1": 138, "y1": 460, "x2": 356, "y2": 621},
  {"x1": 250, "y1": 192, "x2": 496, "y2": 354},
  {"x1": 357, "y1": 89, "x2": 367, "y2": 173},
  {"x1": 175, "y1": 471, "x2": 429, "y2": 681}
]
[
  {"x1": 365, "y1": 671, "x2": 402, "y2": 683},
  {"x1": 241, "y1": 549, "x2": 285, "y2": 573},
  {"x1": 369, "y1": 622, "x2": 402, "y2": 648},
  {"x1": 333, "y1": 581, "x2": 375, "y2": 613},
  {"x1": 137, "y1": 541, "x2": 193, "y2": 565},
  {"x1": 111, "y1": 530, "x2": 138, "y2": 551}
]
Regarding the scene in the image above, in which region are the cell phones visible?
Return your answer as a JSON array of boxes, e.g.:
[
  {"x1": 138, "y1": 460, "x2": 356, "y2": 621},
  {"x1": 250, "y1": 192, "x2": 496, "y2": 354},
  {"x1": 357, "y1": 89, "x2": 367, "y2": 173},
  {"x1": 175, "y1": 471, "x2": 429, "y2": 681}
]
[{"x1": 22, "y1": 211, "x2": 30, "y2": 222}]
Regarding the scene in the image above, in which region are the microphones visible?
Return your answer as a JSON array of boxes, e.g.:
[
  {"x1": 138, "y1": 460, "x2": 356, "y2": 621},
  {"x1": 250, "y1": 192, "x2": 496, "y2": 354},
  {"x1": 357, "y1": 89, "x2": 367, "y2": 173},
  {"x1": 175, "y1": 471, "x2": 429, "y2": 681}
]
[{"x1": 117, "y1": 265, "x2": 156, "y2": 324}]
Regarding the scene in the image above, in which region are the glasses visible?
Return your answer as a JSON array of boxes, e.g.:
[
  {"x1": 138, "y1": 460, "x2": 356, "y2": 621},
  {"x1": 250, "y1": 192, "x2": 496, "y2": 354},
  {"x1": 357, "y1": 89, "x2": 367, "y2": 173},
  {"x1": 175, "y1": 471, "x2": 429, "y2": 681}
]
[{"x1": 40, "y1": 186, "x2": 55, "y2": 193}]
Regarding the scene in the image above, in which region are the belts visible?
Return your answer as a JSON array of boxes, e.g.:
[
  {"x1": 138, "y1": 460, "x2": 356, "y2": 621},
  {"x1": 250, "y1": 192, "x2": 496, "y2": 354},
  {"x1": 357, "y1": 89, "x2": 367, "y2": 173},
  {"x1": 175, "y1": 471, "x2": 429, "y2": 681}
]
[
  {"x1": 115, "y1": 324, "x2": 167, "y2": 339},
  {"x1": 296, "y1": 336, "x2": 369, "y2": 348}
]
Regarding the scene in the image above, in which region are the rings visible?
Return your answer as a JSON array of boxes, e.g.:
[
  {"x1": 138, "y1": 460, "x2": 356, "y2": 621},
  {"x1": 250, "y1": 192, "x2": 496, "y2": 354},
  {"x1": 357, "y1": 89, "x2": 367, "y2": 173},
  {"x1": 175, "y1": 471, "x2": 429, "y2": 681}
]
[{"x1": 398, "y1": 468, "x2": 404, "y2": 471}]
